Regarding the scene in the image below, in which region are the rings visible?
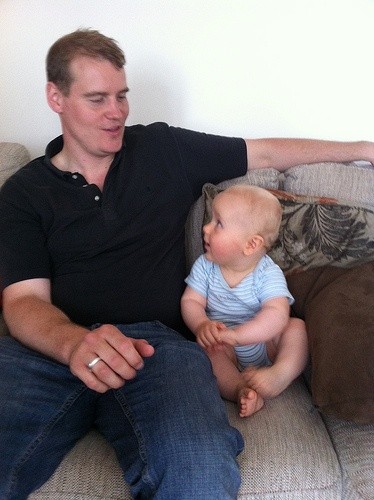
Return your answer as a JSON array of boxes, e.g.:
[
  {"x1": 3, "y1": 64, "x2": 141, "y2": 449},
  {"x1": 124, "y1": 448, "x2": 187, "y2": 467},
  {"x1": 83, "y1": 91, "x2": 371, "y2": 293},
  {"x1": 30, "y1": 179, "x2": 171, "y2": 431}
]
[{"x1": 87, "y1": 356, "x2": 101, "y2": 370}]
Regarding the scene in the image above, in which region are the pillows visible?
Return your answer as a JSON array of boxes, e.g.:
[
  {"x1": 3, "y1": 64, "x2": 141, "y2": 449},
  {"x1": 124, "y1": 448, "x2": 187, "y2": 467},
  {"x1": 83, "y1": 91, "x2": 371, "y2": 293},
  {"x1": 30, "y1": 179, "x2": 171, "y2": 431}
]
[
  {"x1": 286, "y1": 261, "x2": 373, "y2": 422},
  {"x1": 267, "y1": 187, "x2": 374, "y2": 278}
]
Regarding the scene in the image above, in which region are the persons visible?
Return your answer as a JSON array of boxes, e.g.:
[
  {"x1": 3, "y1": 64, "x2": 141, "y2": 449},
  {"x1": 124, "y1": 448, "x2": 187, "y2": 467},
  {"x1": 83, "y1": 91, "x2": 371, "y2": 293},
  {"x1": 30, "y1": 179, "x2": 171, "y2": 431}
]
[
  {"x1": 0, "y1": 25, "x2": 373, "y2": 500},
  {"x1": 181, "y1": 184, "x2": 310, "y2": 418}
]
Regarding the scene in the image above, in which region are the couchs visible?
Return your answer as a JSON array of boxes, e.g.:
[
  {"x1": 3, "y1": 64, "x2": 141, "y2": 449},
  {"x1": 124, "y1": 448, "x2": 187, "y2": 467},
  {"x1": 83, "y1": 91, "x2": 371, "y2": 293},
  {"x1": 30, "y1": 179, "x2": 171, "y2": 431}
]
[{"x1": 1, "y1": 142, "x2": 373, "y2": 500}]
